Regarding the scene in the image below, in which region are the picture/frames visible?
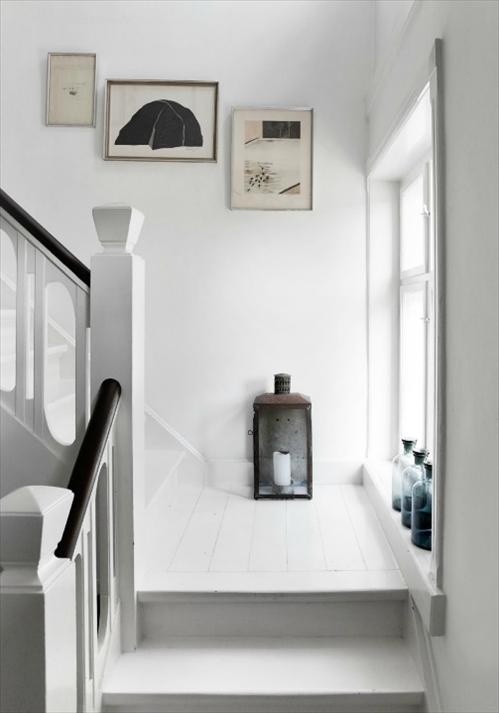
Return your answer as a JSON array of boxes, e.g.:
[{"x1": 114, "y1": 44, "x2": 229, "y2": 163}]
[
  {"x1": 45, "y1": 52, "x2": 98, "y2": 128},
  {"x1": 229, "y1": 108, "x2": 314, "y2": 211},
  {"x1": 103, "y1": 80, "x2": 219, "y2": 162}
]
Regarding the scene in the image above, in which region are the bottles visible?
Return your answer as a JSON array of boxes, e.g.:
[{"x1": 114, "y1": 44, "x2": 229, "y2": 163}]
[{"x1": 391, "y1": 437, "x2": 432, "y2": 551}]
